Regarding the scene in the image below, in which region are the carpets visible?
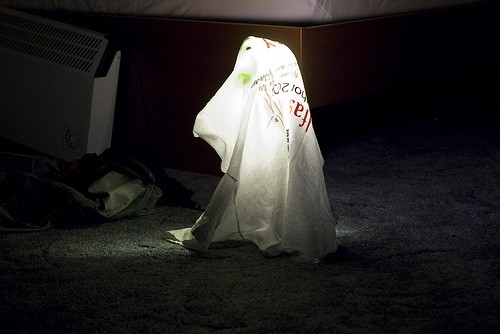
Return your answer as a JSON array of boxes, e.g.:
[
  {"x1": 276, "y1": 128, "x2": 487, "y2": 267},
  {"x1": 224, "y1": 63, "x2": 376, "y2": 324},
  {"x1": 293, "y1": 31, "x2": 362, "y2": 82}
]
[{"x1": 1, "y1": 139, "x2": 498, "y2": 334}]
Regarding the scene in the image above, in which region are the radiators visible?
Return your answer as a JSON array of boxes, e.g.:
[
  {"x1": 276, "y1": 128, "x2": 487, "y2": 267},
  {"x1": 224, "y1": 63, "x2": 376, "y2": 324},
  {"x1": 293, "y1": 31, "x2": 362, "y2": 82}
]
[{"x1": 0, "y1": 6, "x2": 121, "y2": 176}]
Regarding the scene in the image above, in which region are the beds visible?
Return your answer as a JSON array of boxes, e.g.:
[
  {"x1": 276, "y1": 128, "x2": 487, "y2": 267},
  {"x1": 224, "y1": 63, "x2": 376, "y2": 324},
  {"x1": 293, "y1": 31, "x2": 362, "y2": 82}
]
[{"x1": 0, "y1": 1, "x2": 499, "y2": 176}]
